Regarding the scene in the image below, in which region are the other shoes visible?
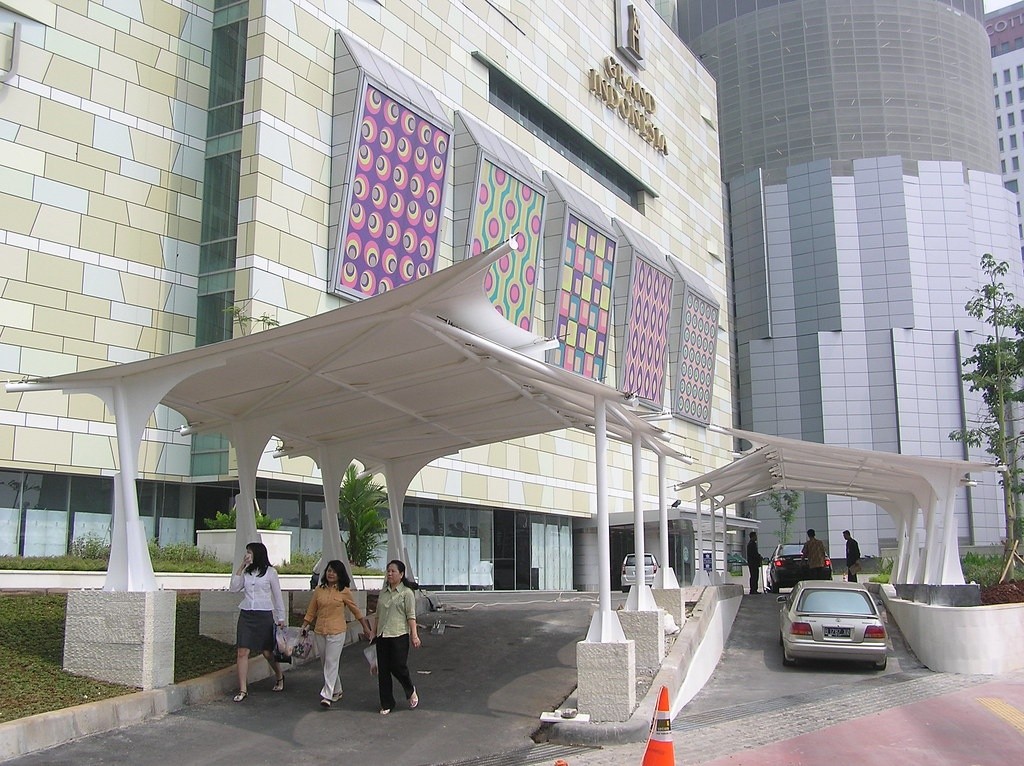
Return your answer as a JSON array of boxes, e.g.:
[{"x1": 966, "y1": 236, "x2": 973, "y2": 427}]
[{"x1": 750, "y1": 589, "x2": 761, "y2": 594}]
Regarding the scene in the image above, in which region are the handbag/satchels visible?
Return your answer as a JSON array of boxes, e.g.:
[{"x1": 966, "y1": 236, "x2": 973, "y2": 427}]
[
  {"x1": 293, "y1": 629, "x2": 313, "y2": 659},
  {"x1": 363, "y1": 644, "x2": 378, "y2": 676},
  {"x1": 272, "y1": 623, "x2": 292, "y2": 665}
]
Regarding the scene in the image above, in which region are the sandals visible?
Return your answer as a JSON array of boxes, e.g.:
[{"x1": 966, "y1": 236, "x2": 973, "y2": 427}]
[
  {"x1": 273, "y1": 675, "x2": 285, "y2": 692},
  {"x1": 233, "y1": 691, "x2": 248, "y2": 702},
  {"x1": 409, "y1": 685, "x2": 419, "y2": 709},
  {"x1": 380, "y1": 708, "x2": 390, "y2": 714}
]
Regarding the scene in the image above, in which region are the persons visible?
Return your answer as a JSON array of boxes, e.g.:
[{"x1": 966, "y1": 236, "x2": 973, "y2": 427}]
[
  {"x1": 747, "y1": 532, "x2": 763, "y2": 594},
  {"x1": 843, "y1": 530, "x2": 860, "y2": 583},
  {"x1": 800, "y1": 529, "x2": 827, "y2": 580},
  {"x1": 230, "y1": 543, "x2": 285, "y2": 702},
  {"x1": 374, "y1": 560, "x2": 421, "y2": 715},
  {"x1": 299, "y1": 560, "x2": 371, "y2": 707}
]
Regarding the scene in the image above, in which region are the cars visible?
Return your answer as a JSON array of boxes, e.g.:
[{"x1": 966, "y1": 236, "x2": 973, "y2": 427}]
[
  {"x1": 776, "y1": 580, "x2": 890, "y2": 670},
  {"x1": 762, "y1": 542, "x2": 835, "y2": 594}
]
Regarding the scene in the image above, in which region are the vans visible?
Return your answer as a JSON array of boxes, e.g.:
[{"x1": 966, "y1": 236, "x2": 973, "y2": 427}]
[{"x1": 620, "y1": 553, "x2": 662, "y2": 593}]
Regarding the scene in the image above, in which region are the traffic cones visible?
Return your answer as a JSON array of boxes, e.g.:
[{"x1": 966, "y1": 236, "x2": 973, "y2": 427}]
[{"x1": 642, "y1": 686, "x2": 674, "y2": 766}]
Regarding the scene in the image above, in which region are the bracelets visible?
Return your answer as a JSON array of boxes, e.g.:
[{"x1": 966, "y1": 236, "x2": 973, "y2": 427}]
[{"x1": 301, "y1": 625, "x2": 305, "y2": 628}]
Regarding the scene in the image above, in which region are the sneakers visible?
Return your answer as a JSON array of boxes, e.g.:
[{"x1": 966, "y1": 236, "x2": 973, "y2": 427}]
[
  {"x1": 331, "y1": 693, "x2": 343, "y2": 702},
  {"x1": 321, "y1": 698, "x2": 331, "y2": 706}
]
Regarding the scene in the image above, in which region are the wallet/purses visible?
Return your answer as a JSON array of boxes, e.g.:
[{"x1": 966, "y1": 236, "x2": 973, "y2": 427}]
[{"x1": 303, "y1": 624, "x2": 310, "y2": 636}]
